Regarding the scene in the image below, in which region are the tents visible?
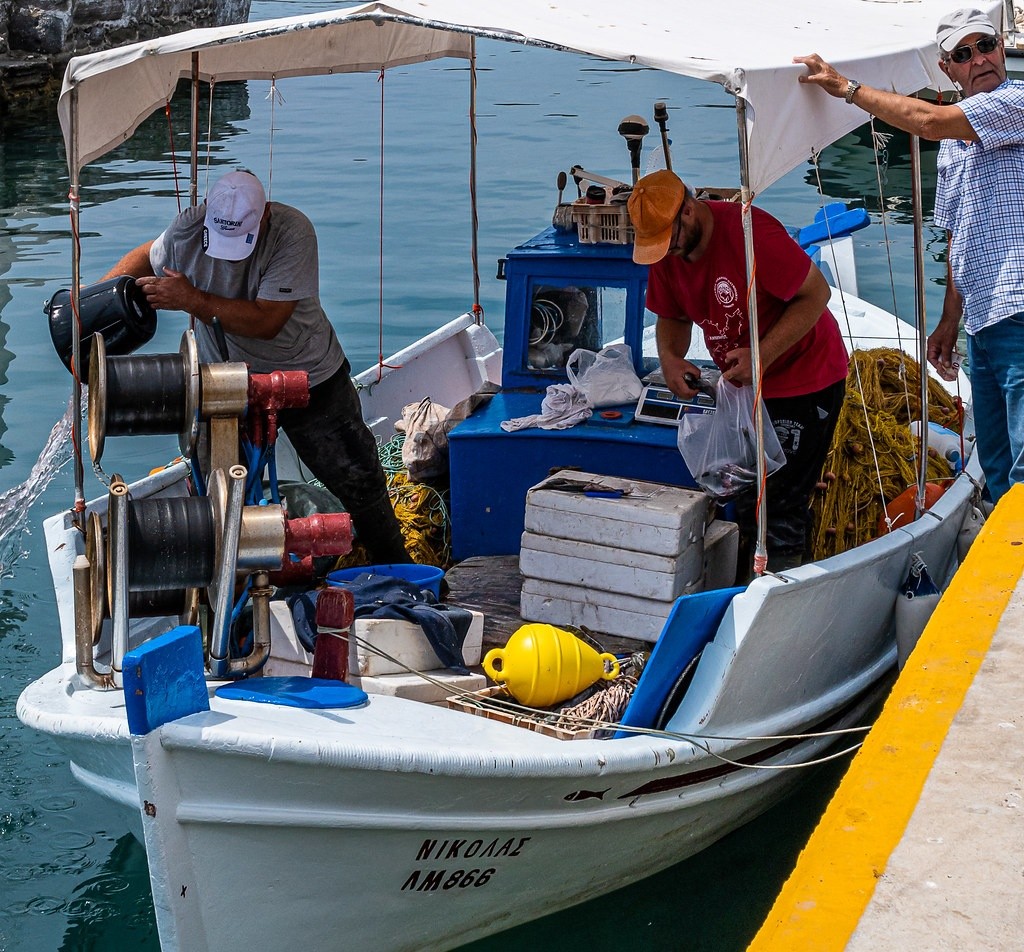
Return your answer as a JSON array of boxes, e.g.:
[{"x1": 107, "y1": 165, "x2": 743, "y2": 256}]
[{"x1": 49, "y1": 0, "x2": 1020, "y2": 603}]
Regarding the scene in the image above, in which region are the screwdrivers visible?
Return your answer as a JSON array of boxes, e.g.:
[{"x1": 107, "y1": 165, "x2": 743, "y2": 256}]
[
  {"x1": 550, "y1": 491, "x2": 652, "y2": 499},
  {"x1": 573, "y1": 165, "x2": 584, "y2": 198},
  {"x1": 557, "y1": 172, "x2": 567, "y2": 208}
]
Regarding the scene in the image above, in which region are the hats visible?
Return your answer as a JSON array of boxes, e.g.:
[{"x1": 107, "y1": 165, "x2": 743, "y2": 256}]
[
  {"x1": 202, "y1": 173, "x2": 268, "y2": 261},
  {"x1": 935, "y1": 8, "x2": 999, "y2": 55},
  {"x1": 626, "y1": 169, "x2": 683, "y2": 264}
]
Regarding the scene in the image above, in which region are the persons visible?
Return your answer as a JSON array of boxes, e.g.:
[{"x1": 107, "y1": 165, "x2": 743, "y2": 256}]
[
  {"x1": 621, "y1": 164, "x2": 856, "y2": 576},
  {"x1": 87, "y1": 167, "x2": 454, "y2": 599},
  {"x1": 786, "y1": 5, "x2": 1024, "y2": 510}
]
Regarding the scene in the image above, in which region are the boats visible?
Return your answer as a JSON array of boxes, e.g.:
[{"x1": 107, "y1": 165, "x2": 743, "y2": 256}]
[{"x1": 13, "y1": 0, "x2": 1024, "y2": 950}]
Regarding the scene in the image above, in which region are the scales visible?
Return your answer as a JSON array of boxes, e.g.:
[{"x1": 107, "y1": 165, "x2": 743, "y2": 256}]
[{"x1": 634, "y1": 365, "x2": 735, "y2": 427}]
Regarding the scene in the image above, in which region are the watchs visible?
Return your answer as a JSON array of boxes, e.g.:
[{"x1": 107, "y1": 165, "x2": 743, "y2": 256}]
[{"x1": 844, "y1": 78, "x2": 862, "y2": 106}]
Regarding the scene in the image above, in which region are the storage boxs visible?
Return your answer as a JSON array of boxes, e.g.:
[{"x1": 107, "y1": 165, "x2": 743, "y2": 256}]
[
  {"x1": 520, "y1": 578, "x2": 704, "y2": 642},
  {"x1": 518, "y1": 531, "x2": 705, "y2": 603},
  {"x1": 524, "y1": 469, "x2": 712, "y2": 558},
  {"x1": 704, "y1": 519, "x2": 740, "y2": 591}
]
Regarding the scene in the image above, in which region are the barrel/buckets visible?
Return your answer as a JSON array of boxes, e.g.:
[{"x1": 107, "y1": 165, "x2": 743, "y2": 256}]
[{"x1": 325, "y1": 562, "x2": 440, "y2": 602}]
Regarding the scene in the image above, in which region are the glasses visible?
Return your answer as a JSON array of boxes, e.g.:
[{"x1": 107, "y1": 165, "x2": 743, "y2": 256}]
[{"x1": 950, "y1": 36, "x2": 998, "y2": 63}]
